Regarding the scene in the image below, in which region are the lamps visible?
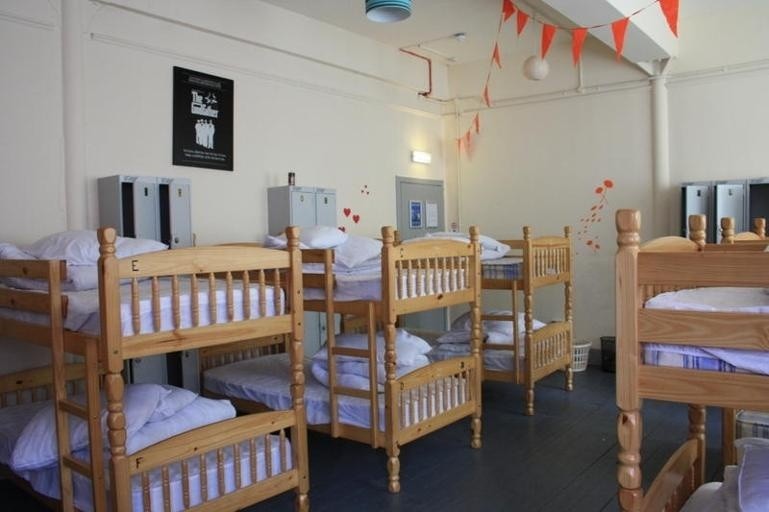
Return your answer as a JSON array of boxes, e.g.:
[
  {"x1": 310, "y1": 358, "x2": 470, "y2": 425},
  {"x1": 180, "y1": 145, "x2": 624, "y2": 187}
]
[
  {"x1": 363, "y1": 0, "x2": 417, "y2": 24},
  {"x1": 522, "y1": 10, "x2": 551, "y2": 82}
]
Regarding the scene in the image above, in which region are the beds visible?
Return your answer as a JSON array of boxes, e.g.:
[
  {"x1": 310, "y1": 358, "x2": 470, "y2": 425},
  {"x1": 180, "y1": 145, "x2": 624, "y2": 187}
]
[
  {"x1": 614, "y1": 208, "x2": 769, "y2": 512},
  {"x1": 0, "y1": 225, "x2": 311, "y2": 512},
  {"x1": 193, "y1": 224, "x2": 484, "y2": 494},
  {"x1": 720, "y1": 217, "x2": 769, "y2": 481},
  {"x1": 338, "y1": 225, "x2": 574, "y2": 416}
]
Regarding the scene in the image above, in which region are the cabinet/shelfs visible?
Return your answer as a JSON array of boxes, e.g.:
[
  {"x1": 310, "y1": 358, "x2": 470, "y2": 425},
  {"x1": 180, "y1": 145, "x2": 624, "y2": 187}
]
[
  {"x1": 267, "y1": 186, "x2": 341, "y2": 358},
  {"x1": 96, "y1": 174, "x2": 201, "y2": 394}
]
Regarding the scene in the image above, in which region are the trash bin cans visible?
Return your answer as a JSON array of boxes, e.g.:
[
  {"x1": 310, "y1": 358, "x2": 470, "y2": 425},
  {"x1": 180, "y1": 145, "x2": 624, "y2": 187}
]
[{"x1": 600, "y1": 336, "x2": 616, "y2": 374}]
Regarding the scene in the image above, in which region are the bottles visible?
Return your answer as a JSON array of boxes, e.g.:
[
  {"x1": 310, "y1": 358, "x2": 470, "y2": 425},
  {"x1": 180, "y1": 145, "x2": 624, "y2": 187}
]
[{"x1": 288, "y1": 171, "x2": 296, "y2": 186}]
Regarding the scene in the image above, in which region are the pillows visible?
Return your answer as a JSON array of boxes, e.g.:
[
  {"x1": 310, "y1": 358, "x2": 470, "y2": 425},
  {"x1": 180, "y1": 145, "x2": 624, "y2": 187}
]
[
  {"x1": 731, "y1": 437, "x2": 769, "y2": 512},
  {"x1": 441, "y1": 309, "x2": 546, "y2": 339},
  {"x1": 274, "y1": 224, "x2": 349, "y2": 251},
  {"x1": 312, "y1": 333, "x2": 414, "y2": 367},
  {"x1": 10, "y1": 383, "x2": 172, "y2": 473},
  {"x1": 19, "y1": 227, "x2": 169, "y2": 266},
  {"x1": 147, "y1": 386, "x2": 193, "y2": 424}
]
